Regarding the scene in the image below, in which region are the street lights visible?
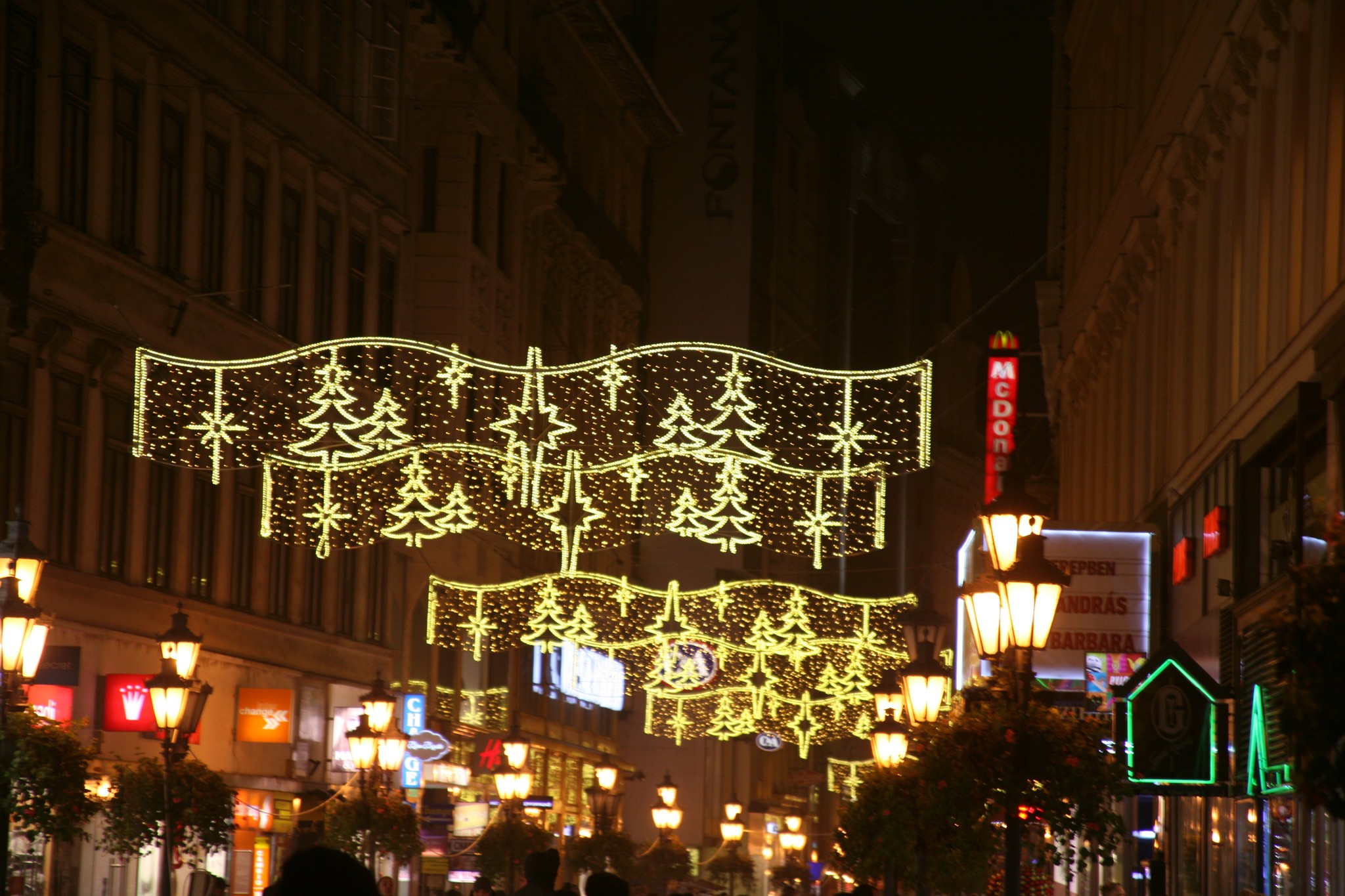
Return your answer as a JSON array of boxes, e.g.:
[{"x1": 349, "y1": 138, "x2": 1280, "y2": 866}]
[
  {"x1": 343, "y1": 669, "x2": 819, "y2": 895},
  {"x1": 871, "y1": 583, "x2": 955, "y2": 894},
  {"x1": 143, "y1": 599, "x2": 215, "y2": 895},
  {"x1": 0, "y1": 497, "x2": 51, "y2": 895},
  {"x1": 956, "y1": 464, "x2": 1068, "y2": 896}
]
